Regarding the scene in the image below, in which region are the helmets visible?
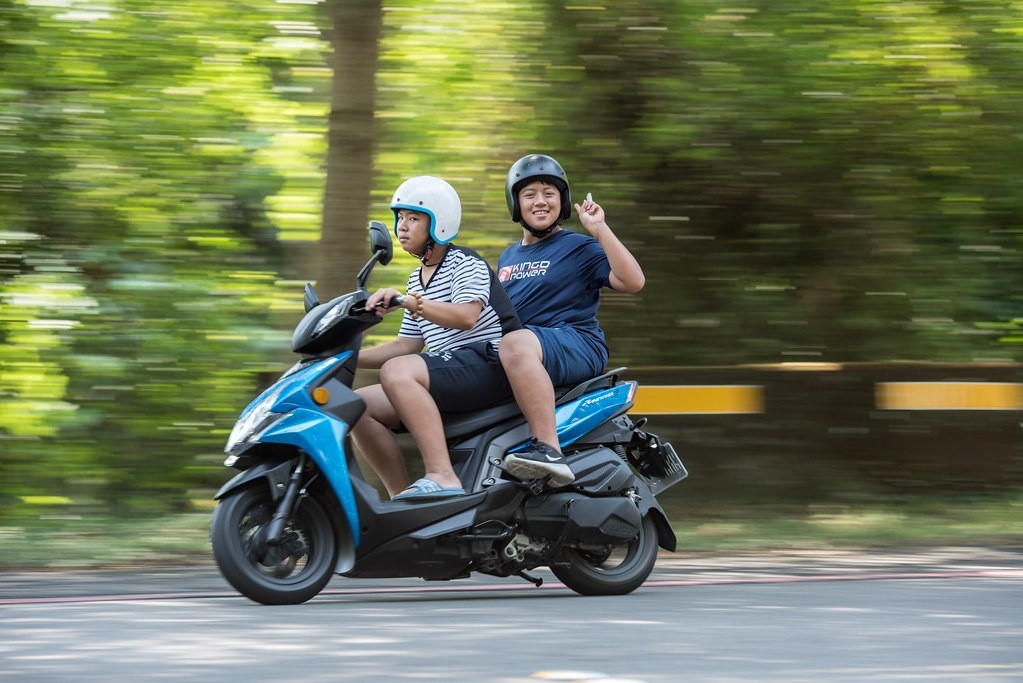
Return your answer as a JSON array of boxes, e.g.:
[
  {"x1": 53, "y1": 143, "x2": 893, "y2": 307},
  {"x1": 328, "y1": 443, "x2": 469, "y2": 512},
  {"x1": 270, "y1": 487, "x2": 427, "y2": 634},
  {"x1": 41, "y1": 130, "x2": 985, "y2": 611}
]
[
  {"x1": 390, "y1": 176, "x2": 462, "y2": 245},
  {"x1": 506, "y1": 153, "x2": 571, "y2": 223}
]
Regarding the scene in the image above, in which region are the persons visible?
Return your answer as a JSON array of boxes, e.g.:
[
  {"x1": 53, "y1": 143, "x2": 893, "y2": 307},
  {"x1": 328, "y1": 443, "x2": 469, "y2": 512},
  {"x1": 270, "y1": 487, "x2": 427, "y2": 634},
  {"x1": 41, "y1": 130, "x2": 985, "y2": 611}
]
[
  {"x1": 495, "y1": 154, "x2": 645, "y2": 487},
  {"x1": 350, "y1": 175, "x2": 523, "y2": 501}
]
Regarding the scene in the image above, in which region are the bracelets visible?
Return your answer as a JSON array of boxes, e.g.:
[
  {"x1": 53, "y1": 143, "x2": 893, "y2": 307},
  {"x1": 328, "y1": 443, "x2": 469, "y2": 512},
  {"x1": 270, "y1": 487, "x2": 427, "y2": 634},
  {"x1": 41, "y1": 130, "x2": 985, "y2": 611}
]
[{"x1": 406, "y1": 292, "x2": 424, "y2": 320}]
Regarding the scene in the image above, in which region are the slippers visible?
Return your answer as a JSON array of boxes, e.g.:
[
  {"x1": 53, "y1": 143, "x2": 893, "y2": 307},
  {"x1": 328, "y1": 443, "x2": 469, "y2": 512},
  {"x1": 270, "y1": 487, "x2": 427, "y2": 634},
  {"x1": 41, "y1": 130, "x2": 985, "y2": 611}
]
[{"x1": 391, "y1": 479, "x2": 466, "y2": 499}]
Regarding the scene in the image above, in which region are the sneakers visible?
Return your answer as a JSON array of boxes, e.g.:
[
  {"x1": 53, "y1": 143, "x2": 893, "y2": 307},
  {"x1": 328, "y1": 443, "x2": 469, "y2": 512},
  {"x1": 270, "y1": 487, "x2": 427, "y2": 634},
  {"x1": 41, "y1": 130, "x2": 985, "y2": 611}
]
[{"x1": 503, "y1": 436, "x2": 576, "y2": 487}]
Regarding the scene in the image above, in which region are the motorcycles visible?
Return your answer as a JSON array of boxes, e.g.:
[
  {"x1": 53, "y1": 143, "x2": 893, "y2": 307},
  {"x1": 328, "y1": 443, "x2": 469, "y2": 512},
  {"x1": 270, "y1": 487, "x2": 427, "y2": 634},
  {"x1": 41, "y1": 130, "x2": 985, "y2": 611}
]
[{"x1": 209, "y1": 220, "x2": 687, "y2": 606}]
